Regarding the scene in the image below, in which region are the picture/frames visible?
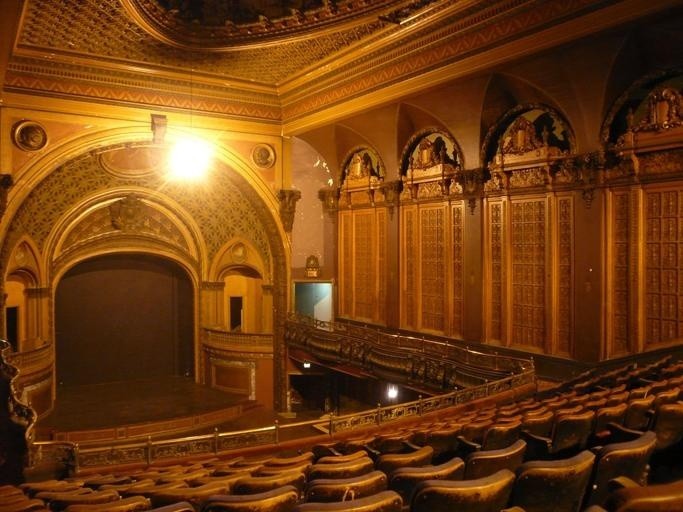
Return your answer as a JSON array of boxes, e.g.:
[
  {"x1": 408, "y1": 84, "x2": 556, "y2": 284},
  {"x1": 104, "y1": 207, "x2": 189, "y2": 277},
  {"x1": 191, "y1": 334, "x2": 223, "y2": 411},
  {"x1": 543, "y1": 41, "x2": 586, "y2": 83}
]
[
  {"x1": 14, "y1": 121, "x2": 49, "y2": 152},
  {"x1": 250, "y1": 143, "x2": 276, "y2": 169}
]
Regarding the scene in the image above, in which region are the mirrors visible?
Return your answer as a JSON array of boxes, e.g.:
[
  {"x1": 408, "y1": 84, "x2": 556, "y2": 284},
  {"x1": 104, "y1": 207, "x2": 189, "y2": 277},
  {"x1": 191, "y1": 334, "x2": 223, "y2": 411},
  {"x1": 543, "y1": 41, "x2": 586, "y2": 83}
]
[{"x1": 293, "y1": 279, "x2": 335, "y2": 325}]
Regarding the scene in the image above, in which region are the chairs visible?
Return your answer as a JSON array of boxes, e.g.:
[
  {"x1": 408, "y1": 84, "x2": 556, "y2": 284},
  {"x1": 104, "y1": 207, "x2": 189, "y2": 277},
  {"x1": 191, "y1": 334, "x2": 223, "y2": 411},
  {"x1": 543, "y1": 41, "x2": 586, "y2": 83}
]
[{"x1": 1, "y1": 354, "x2": 682, "y2": 512}]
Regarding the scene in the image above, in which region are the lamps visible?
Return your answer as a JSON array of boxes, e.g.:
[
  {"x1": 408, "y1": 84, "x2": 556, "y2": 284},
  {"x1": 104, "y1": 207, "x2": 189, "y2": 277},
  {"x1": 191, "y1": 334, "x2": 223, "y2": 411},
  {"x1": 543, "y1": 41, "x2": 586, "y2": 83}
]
[{"x1": 170, "y1": 69, "x2": 211, "y2": 171}]
